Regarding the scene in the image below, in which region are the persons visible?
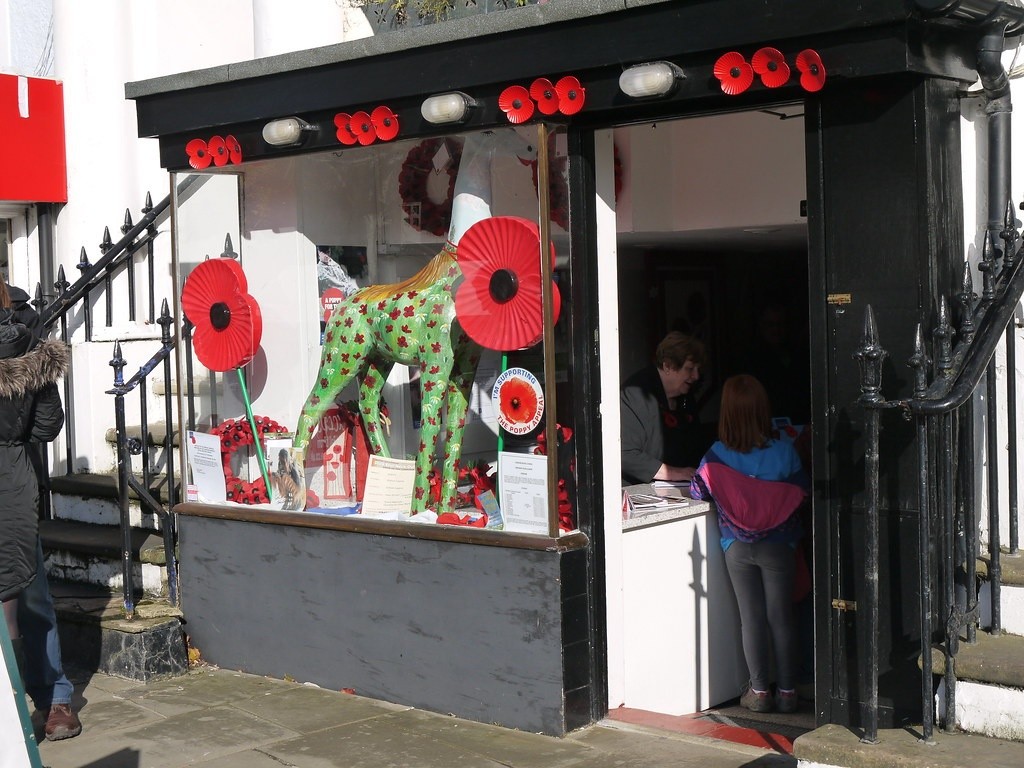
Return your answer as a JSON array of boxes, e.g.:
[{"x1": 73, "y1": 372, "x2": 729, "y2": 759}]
[
  {"x1": 0, "y1": 271, "x2": 82, "y2": 742},
  {"x1": 620, "y1": 330, "x2": 706, "y2": 484},
  {"x1": 690, "y1": 374, "x2": 809, "y2": 713}
]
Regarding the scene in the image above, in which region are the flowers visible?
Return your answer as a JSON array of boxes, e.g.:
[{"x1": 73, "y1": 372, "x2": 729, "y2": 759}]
[
  {"x1": 336, "y1": 396, "x2": 578, "y2": 531},
  {"x1": 208, "y1": 415, "x2": 287, "y2": 505}
]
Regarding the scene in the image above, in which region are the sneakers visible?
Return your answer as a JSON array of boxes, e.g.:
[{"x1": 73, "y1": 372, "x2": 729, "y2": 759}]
[
  {"x1": 41, "y1": 704, "x2": 82, "y2": 741},
  {"x1": 776, "y1": 689, "x2": 797, "y2": 712},
  {"x1": 740, "y1": 681, "x2": 772, "y2": 712}
]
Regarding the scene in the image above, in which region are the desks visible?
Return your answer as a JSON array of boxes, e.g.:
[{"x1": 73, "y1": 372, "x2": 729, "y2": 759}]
[{"x1": 621, "y1": 479, "x2": 777, "y2": 717}]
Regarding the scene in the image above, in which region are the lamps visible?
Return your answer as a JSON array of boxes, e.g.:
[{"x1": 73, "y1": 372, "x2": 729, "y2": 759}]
[
  {"x1": 262, "y1": 116, "x2": 312, "y2": 149},
  {"x1": 614, "y1": 60, "x2": 687, "y2": 103},
  {"x1": 420, "y1": 91, "x2": 476, "y2": 128}
]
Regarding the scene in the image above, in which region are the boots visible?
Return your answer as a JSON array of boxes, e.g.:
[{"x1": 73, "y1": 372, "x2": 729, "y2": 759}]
[{"x1": 11, "y1": 635, "x2": 26, "y2": 698}]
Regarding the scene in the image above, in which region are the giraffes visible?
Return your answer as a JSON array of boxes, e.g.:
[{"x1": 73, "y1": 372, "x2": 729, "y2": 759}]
[{"x1": 293, "y1": 127, "x2": 537, "y2": 517}]
